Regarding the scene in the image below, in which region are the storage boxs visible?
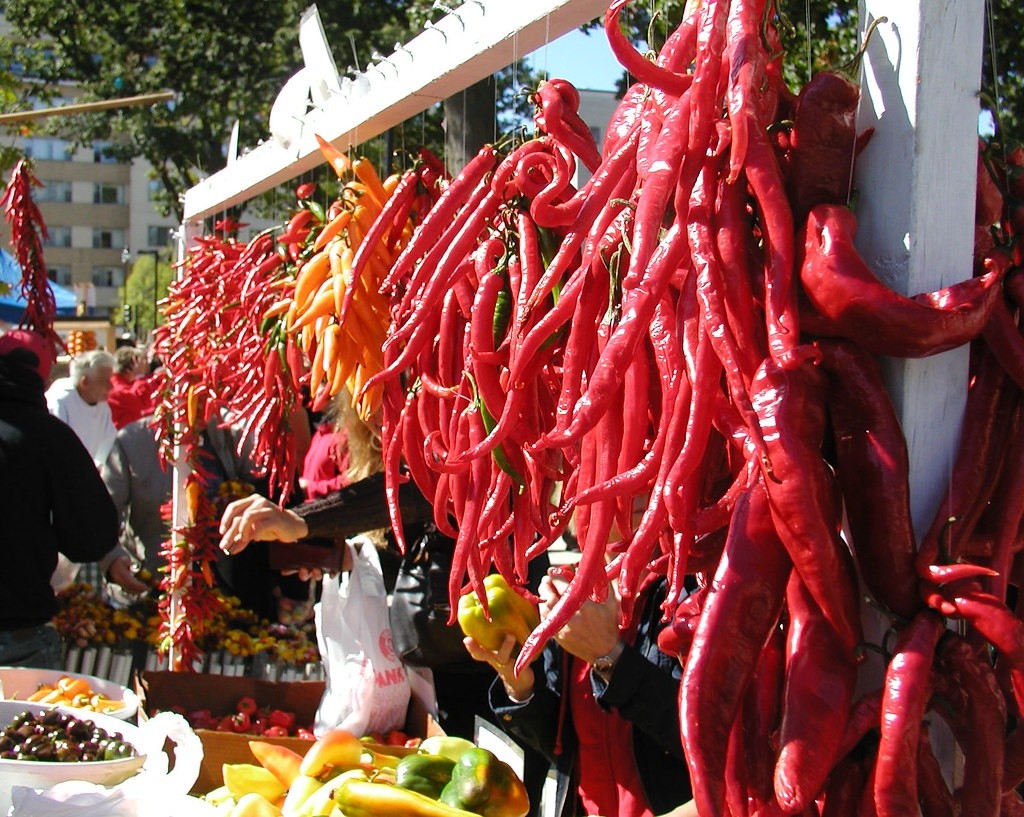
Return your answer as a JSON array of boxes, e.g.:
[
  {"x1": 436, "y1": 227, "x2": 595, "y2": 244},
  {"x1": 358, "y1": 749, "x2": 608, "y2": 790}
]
[{"x1": 133, "y1": 669, "x2": 446, "y2": 797}]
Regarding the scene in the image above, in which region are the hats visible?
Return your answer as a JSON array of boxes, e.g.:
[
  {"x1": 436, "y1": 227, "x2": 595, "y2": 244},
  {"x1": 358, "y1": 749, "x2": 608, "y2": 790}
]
[{"x1": 0, "y1": 330, "x2": 53, "y2": 380}]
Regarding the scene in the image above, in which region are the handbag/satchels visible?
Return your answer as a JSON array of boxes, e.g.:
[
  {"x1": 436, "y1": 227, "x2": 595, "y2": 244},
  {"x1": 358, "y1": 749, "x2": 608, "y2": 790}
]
[
  {"x1": 390, "y1": 525, "x2": 549, "y2": 668},
  {"x1": 313, "y1": 534, "x2": 411, "y2": 743}
]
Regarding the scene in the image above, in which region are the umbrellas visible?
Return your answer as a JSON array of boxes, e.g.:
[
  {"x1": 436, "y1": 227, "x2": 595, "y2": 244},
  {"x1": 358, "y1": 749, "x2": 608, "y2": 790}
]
[{"x1": 0, "y1": 249, "x2": 77, "y2": 326}]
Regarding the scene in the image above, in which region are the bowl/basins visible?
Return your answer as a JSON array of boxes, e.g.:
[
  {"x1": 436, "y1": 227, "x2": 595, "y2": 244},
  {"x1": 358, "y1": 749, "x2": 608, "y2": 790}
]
[
  {"x1": 0, "y1": 666, "x2": 139, "y2": 725},
  {"x1": 0, "y1": 701, "x2": 147, "y2": 817}
]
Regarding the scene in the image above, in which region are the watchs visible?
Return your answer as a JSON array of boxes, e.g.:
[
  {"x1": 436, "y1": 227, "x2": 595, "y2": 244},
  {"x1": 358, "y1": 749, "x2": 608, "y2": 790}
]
[{"x1": 593, "y1": 639, "x2": 624, "y2": 674}]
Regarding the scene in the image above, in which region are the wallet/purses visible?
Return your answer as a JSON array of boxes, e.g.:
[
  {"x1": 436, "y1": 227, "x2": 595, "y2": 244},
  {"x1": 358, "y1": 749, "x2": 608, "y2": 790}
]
[{"x1": 279, "y1": 531, "x2": 346, "y2": 573}]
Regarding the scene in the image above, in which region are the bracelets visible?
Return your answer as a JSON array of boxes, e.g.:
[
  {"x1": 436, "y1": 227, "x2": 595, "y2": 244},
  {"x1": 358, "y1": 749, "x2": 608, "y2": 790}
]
[{"x1": 508, "y1": 694, "x2": 534, "y2": 704}]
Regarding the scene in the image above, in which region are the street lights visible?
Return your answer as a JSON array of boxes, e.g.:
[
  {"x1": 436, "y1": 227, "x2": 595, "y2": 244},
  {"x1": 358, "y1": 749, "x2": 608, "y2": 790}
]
[{"x1": 136, "y1": 249, "x2": 158, "y2": 341}]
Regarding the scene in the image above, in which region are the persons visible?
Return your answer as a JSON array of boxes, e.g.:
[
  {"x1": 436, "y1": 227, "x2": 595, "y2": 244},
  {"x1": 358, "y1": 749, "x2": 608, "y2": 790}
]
[{"x1": 1, "y1": 330, "x2": 697, "y2": 816}]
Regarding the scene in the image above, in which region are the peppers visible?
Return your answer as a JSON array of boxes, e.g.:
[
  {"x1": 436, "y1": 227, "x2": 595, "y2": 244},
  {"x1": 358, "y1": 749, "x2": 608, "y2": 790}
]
[{"x1": 1, "y1": 0, "x2": 1024, "y2": 817}]
